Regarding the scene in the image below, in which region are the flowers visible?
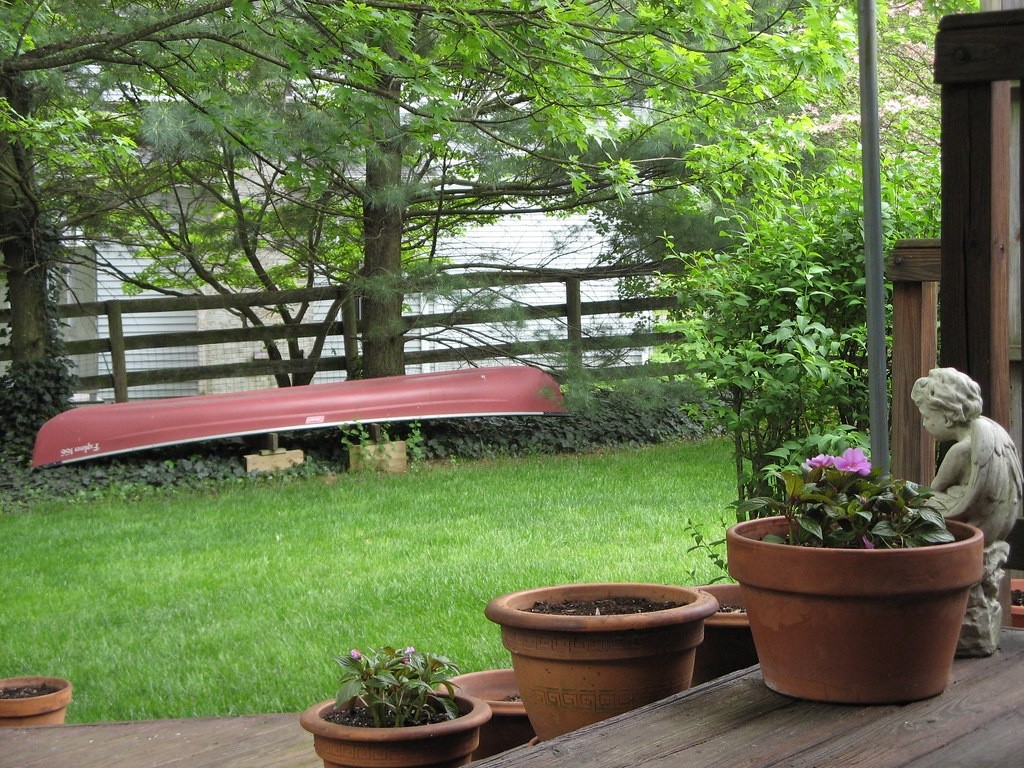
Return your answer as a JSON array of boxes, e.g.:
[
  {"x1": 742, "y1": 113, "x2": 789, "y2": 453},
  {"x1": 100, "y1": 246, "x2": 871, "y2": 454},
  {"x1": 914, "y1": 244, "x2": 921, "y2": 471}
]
[
  {"x1": 734, "y1": 430, "x2": 956, "y2": 547},
  {"x1": 332, "y1": 646, "x2": 463, "y2": 727}
]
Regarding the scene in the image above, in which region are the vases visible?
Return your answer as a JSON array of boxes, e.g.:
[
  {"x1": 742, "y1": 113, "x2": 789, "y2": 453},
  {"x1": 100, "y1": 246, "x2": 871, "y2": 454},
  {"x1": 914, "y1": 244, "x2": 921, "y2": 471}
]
[
  {"x1": 0, "y1": 677, "x2": 72, "y2": 727},
  {"x1": 438, "y1": 668, "x2": 538, "y2": 762},
  {"x1": 690, "y1": 585, "x2": 760, "y2": 689},
  {"x1": 727, "y1": 515, "x2": 984, "y2": 705},
  {"x1": 300, "y1": 692, "x2": 492, "y2": 768},
  {"x1": 483, "y1": 582, "x2": 719, "y2": 751}
]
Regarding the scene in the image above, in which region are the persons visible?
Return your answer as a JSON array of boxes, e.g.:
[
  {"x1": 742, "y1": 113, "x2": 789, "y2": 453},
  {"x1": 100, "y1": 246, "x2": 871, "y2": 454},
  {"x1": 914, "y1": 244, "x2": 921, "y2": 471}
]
[{"x1": 911, "y1": 368, "x2": 1024, "y2": 545}]
